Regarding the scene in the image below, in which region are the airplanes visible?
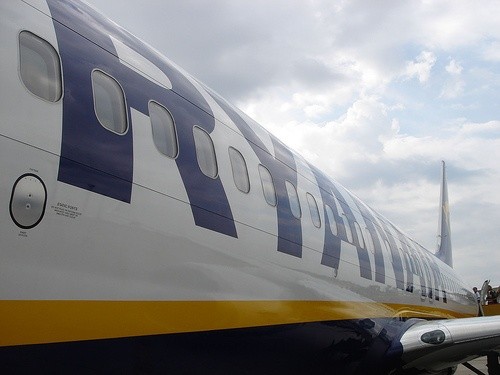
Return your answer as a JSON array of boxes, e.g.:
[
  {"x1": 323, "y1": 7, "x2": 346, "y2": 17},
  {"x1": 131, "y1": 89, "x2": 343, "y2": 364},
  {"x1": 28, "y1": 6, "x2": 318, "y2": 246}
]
[{"x1": 1, "y1": 1, "x2": 499, "y2": 375}]
